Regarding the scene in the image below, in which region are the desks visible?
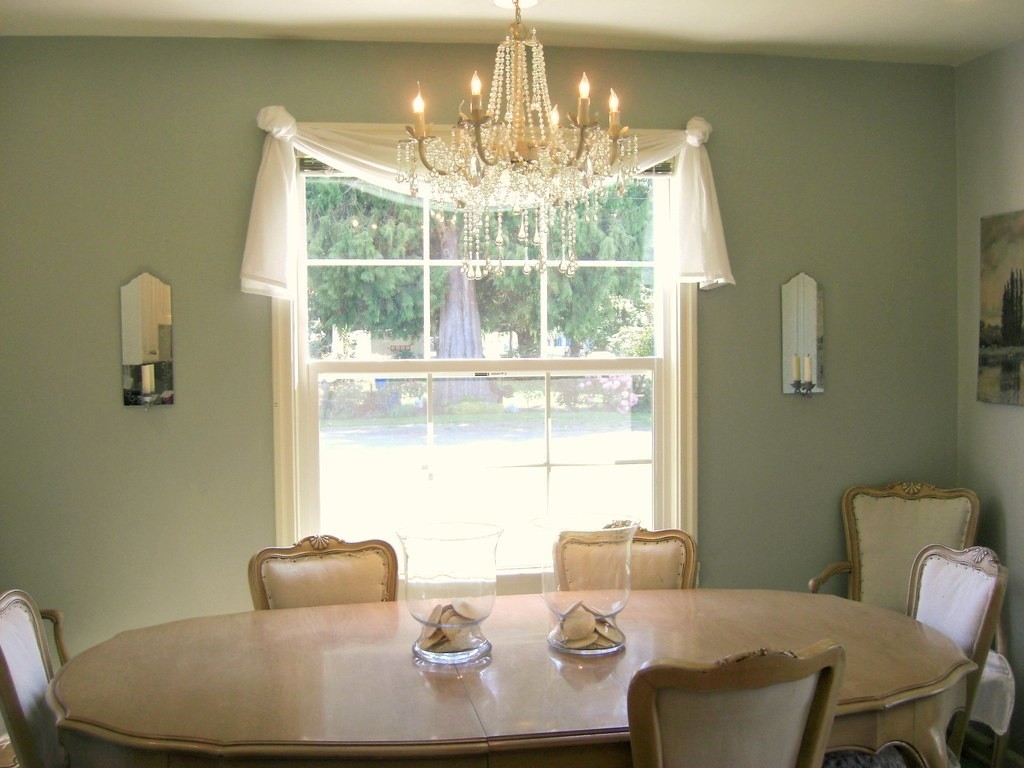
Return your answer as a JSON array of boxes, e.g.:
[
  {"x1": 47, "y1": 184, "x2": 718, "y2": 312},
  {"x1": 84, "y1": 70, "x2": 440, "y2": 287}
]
[{"x1": 43, "y1": 585, "x2": 980, "y2": 768}]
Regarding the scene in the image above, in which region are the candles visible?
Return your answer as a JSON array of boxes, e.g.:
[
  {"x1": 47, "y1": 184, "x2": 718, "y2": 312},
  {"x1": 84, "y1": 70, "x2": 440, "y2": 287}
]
[
  {"x1": 149, "y1": 364, "x2": 156, "y2": 393},
  {"x1": 792, "y1": 352, "x2": 801, "y2": 381},
  {"x1": 804, "y1": 353, "x2": 814, "y2": 383},
  {"x1": 140, "y1": 364, "x2": 151, "y2": 395}
]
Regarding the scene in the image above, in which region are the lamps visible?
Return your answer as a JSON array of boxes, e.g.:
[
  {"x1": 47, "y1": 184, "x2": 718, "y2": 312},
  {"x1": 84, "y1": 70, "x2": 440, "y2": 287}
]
[{"x1": 394, "y1": 0, "x2": 641, "y2": 281}]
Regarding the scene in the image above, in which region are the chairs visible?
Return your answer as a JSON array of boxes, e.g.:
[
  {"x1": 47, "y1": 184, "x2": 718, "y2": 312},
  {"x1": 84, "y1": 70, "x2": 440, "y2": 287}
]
[
  {"x1": 556, "y1": 520, "x2": 706, "y2": 593},
  {"x1": 241, "y1": 533, "x2": 405, "y2": 612},
  {"x1": 624, "y1": 639, "x2": 851, "y2": 768},
  {"x1": 0, "y1": 584, "x2": 74, "y2": 768},
  {"x1": 909, "y1": 544, "x2": 1010, "y2": 768},
  {"x1": 811, "y1": 477, "x2": 1014, "y2": 768}
]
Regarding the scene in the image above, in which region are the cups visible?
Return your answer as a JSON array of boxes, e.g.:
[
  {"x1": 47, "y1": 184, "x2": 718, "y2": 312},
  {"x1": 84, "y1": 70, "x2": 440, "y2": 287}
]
[
  {"x1": 396, "y1": 520, "x2": 503, "y2": 665},
  {"x1": 532, "y1": 511, "x2": 641, "y2": 654}
]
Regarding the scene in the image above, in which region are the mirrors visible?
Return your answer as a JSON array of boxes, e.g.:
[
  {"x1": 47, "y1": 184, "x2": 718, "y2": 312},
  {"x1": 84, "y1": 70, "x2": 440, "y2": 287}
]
[
  {"x1": 121, "y1": 271, "x2": 174, "y2": 406},
  {"x1": 781, "y1": 273, "x2": 826, "y2": 395}
]
